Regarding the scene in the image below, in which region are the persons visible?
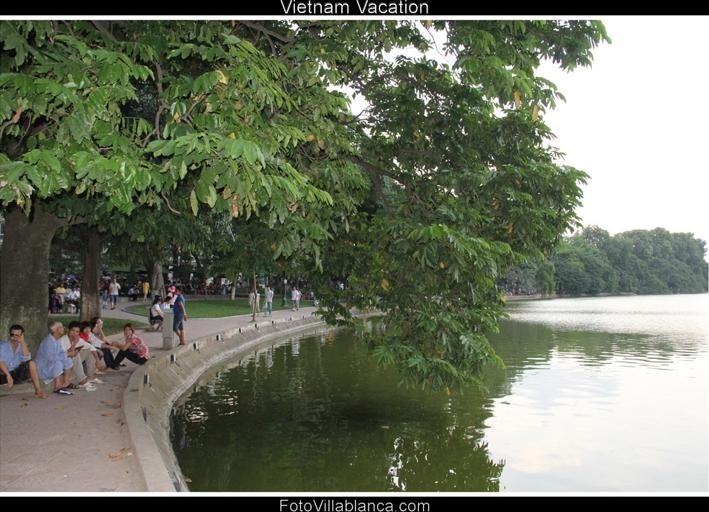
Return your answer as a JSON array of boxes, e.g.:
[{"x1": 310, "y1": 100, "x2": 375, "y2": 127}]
[
  {"x1": 291, "y1": 285, "x2": 302, "y2": 311},
  {"x1": 264, "y1": 285, "x2": 273, "y2": 317},
  {"x1": 248, "y1": 288, "x2": 260, "y2": 313},
  {"x1": 0, "y1": 324, "x2": 49, "y2": 399},
  {"x1": 34, "y1": 274, "x2": 232, "y2": 395}
]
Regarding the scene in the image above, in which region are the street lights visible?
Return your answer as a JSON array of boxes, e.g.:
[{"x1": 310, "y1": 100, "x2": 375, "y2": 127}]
[{"x1": 282, "y1": 278, "x2": 289, "y2": 305}]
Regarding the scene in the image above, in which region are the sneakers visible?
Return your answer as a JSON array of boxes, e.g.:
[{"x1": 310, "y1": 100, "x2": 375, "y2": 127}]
[{"x1": 55, "y1": 369, "x2": 105, "y2": 395}]
